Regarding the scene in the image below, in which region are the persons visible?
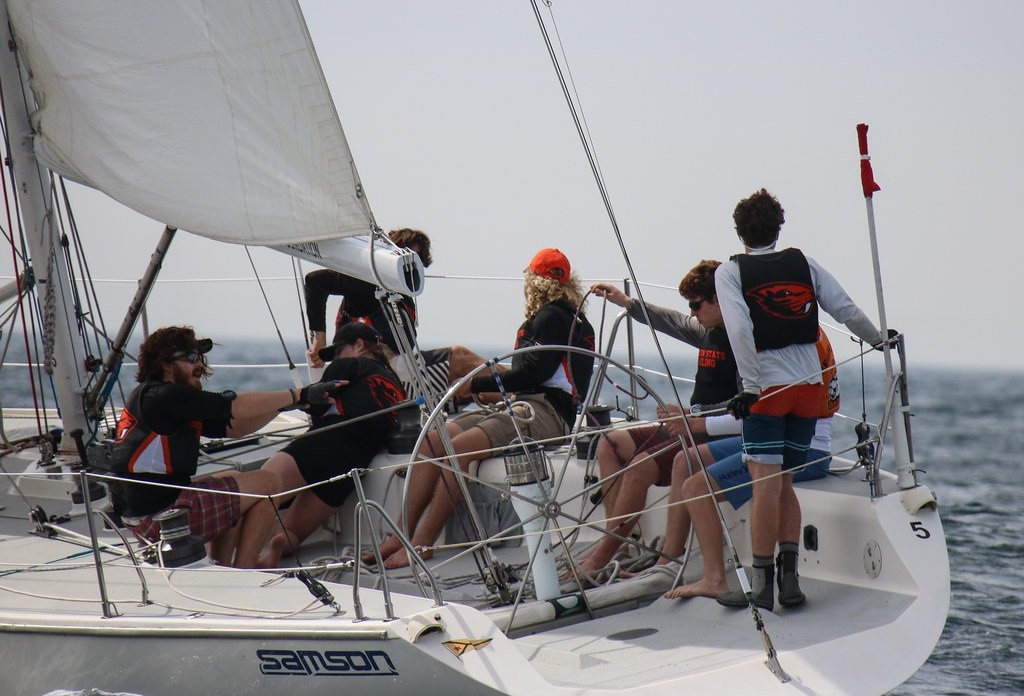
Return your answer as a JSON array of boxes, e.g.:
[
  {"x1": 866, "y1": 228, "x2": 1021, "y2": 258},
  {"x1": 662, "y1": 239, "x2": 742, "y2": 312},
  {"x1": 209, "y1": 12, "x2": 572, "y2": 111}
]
[
  {"x1": 558, "y1": 261, "x2": 739, "y2": 581},
  {"x1": 303, "y1": 228, "x2": 514, "y2": 411},
  {"x1": 111, "y1": 327, "x2": 349, "y2": 568},
  {"x1": 715, "y1": 188, "x2": 898, "y2": 611},
  {"x1": 619, "y1": 328, "x2": 841, "y2": 600},
  {"x1": 362, "y1": 248, "x2": 595, "y2": 570},
  {"x1": 258, "y1": 322, "x2": 408, "y2": 568}
]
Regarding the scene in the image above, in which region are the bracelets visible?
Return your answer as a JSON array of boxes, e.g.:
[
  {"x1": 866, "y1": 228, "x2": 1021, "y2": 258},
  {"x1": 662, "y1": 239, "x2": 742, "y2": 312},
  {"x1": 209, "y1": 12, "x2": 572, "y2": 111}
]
[{"x1": 282, "y1": 389, "x2": 297, "y2": 411}]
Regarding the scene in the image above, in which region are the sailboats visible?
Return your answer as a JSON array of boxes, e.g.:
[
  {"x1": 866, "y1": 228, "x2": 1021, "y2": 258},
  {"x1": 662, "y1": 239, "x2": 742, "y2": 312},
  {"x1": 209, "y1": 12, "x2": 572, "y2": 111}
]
[{"x1": 0, "y1": 0, "x2": 952, "y2": 696}]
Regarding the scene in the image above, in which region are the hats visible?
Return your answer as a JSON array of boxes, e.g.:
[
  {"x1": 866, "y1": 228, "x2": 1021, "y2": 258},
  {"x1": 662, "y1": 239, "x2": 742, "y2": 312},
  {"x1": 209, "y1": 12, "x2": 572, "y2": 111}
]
[
  {"x1": 523, "y1": 248, "x2": 571, "y2": 282},
  {"x1": 318, "y1": 322, "x2": 379, "y2": 362},
  {"x1": 147, "y1": 338, "x2": 213, "y2": 362}
]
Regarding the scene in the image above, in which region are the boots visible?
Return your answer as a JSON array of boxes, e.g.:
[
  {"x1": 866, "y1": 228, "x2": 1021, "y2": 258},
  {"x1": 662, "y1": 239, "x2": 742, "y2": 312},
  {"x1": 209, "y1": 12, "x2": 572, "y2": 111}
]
[
  {"x1": 715, "y1": 567, "x2": 774, "y2": 611},
  {"x1": 776, "y1": 551, "x2": 806, "y2": 606}
]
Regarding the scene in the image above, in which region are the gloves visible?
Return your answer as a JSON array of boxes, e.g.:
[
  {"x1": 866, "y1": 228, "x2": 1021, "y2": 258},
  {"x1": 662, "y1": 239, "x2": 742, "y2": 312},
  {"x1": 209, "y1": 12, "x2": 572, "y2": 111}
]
[
  {"x1": 726, "y1": 392, "x2": 759, "y2": 420},
  {"x1": 872, "y1": 329, "x2": 900, "y2": 351}
]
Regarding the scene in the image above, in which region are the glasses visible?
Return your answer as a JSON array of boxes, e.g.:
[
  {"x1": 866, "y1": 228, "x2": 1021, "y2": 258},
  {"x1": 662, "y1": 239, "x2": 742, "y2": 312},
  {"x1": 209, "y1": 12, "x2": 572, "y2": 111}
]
[
  {"x1": 689, "y1": 295, "x2": 712, "y2": 312},
  {"x1": 166, "y1": 351, "x2": 209, "y2": 367}
]
